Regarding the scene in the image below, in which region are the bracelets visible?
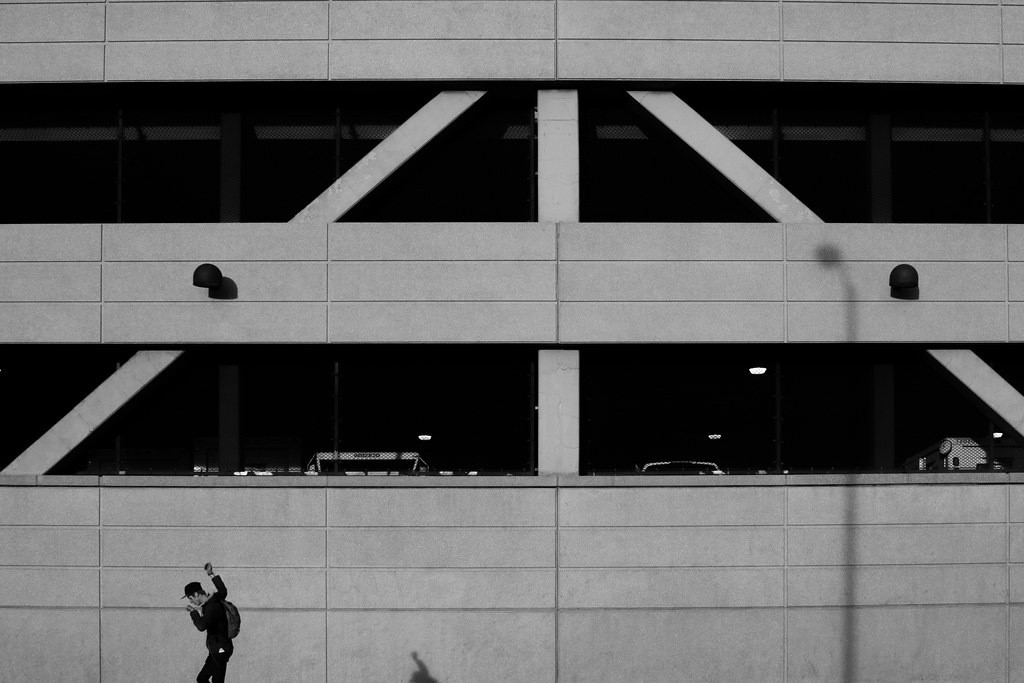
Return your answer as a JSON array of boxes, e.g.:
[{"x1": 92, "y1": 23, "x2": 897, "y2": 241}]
[{"x1": 207, "y1": 572, "x2": 214, "y2": 575}]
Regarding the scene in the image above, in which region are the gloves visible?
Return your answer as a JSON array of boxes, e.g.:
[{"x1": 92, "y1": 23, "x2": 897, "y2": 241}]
[{"x1": 205, "y1": 563, "x2": 213, "y2": 576}]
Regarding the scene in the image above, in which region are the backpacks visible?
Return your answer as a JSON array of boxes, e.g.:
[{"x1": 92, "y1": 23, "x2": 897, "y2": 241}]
[{"x1": 221, "y1": 601, "x2": 241, "y2": 640}]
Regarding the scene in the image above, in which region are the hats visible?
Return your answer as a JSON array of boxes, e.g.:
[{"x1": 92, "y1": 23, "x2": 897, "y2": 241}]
[{"x1": 180, "y1": 582, "x2": 202, "y2": 600}]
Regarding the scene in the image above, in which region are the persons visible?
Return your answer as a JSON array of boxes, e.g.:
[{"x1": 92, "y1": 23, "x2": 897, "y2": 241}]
[{"x1": 181, "y1": 561, "x2": 235, "y2": 682}]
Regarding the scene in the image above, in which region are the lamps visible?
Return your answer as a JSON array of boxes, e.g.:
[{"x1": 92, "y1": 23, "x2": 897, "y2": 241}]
[
  {"x1": 748, "y1": 343, "x2": 767, "y2": 374},
  {"x1": 709, "y1": 434, "x2": 722, "y2": 439},
  {"x1": 418, "y1": 434, "x2": 431, "y2": 440},
  {"x1": 993, "y1": 433, "x2": 1004, "y2": 438}
]
